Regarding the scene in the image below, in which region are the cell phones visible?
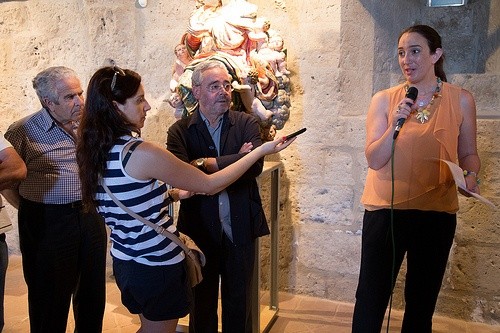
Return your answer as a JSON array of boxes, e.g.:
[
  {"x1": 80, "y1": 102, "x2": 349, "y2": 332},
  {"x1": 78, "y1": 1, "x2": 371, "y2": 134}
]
[{"x1": 286, "y1": 128, "x2": 306, "y2": 140}]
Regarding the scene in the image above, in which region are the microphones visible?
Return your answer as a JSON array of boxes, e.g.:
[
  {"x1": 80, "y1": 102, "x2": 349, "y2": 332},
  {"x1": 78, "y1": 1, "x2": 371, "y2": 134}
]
[{"x1": 392, "y1": 87, "x2": 418, "y2": 139}]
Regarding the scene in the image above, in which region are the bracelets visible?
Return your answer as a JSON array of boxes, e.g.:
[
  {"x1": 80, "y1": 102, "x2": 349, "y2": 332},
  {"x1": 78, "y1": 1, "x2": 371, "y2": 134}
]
[{"x1": 463, "y1": 169, "x2": 481, "y2": 184}]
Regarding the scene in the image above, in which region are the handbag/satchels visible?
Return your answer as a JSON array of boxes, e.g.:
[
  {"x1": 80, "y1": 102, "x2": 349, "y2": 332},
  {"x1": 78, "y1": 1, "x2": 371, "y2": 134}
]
[{"x1": 179, "y1": 231, "x2": 206, "y2": 289}]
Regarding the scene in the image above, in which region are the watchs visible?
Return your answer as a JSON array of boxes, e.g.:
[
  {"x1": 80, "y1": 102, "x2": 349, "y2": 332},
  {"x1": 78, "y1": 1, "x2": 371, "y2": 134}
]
[{"x1": 197, "y1": 158, "x2": 207, "y2": 174}]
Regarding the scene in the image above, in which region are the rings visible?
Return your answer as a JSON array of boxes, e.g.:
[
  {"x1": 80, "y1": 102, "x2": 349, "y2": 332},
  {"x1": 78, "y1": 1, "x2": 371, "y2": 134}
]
[{"x1": 396, "y1": 103, "x2": 403, "y2": 114}]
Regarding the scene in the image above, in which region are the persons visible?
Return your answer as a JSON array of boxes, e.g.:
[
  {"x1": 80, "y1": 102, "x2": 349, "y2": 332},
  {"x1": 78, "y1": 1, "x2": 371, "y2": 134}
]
[
  {"x1": 167, "y1": 59, "x2": 270, "y2": 332},
  {"x1": 0, "y1": 66, "x2": 107, "y2": 333},
  {"x1": 75, "y1": 67, "x2": 295, "y2": 333},
  {"x1": 167, "y1": 0, "x2": 291, "y2": 140},
  {"x1": 0, "y1": 131, "x2": 27, "y2": 333},
  {"x1": 352, "y1": 25, "x2": 482, "y2": 333}
]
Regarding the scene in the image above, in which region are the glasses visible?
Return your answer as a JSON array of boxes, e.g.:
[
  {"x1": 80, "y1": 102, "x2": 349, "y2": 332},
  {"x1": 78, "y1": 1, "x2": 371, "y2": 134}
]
[
  {"x1": 204, "y1": 84, "x2": 235, "y2": 94},
  {"x1": 111, "y1": 66, "x2": 126, "y2": 90}
]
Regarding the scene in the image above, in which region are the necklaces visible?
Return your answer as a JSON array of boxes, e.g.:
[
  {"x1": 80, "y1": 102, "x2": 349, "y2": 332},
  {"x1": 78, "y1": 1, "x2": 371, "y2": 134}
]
[{"x1": 405, "y1": 77, "x2": 443, "y2": 124}]
